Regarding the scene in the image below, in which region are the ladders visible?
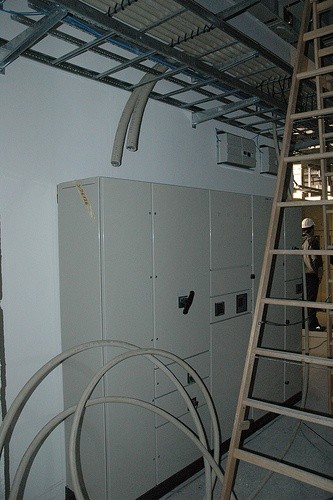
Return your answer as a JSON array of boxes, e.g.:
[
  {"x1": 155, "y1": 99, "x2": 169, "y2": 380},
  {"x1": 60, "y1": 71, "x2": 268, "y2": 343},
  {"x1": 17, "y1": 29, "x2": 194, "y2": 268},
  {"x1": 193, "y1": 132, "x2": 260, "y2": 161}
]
[{"x1": 217, "y1": 0, "x2": 333, "y2": 500}]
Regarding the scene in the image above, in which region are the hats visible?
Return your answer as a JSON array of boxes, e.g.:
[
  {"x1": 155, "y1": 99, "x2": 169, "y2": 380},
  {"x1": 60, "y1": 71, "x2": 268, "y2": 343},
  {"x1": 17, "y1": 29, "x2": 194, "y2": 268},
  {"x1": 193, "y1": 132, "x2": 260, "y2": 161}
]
[{"x1": 302, "y1": 217, "x2": 315, "y2": 229}]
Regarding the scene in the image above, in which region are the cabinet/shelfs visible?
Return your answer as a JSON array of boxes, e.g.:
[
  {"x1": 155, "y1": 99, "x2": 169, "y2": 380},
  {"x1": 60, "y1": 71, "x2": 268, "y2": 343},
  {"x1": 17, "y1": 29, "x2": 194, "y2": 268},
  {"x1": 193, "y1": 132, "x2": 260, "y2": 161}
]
[{"x1": 55, "y1": 176, "x2": 307, "y2": 500}]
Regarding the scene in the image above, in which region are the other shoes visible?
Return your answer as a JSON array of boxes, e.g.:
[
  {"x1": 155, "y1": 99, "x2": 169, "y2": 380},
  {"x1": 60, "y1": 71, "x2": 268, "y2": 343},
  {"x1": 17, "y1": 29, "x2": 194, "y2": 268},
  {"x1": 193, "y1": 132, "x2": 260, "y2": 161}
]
[{"x1": 309, "y1": 324, "x2": 325, "y2": 331}]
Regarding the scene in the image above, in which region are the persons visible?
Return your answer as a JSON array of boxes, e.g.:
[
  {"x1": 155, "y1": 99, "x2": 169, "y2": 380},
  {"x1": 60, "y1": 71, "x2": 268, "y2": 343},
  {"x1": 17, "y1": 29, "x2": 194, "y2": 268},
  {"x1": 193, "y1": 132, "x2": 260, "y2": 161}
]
[{"x1": 302, "y1": 217, "x2": 326, "y2": 332}]
